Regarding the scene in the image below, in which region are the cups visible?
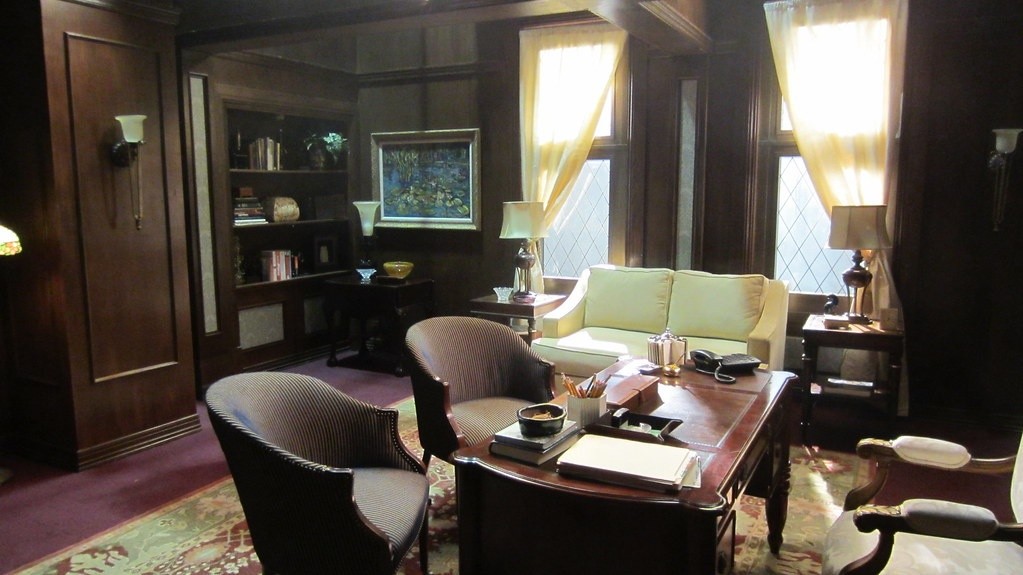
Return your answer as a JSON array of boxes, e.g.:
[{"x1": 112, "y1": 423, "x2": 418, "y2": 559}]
[
  {"x1": 880, "y1": 308, "x2": 899, "y2": 330},
  {"x1": 493, "y1": 286, "x2": 514, "y2": 302},
  {"x1": 356, "y1": 268, "x2": 376, "y2": 285},
  {"x1": 567, "y1": 394, "x2": 606, "y2": 428}
]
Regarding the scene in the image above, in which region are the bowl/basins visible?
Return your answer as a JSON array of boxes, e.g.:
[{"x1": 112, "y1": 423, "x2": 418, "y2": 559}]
[
  {"x1": 517, "y1": 403, "x2": 566, "y2": 438},
  {"x1": 384, "y1": 262, "x2": 414, "y2": 279}
]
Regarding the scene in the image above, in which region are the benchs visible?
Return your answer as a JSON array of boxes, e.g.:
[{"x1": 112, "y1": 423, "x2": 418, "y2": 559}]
[{"x1": 527, "y1": 264, "x2": 792, "y2": 396}]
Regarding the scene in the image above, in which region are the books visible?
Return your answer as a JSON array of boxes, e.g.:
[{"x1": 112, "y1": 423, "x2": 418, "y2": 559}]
[
  {"x1": 248, "y1": 137, "x2": 280, "y2": 171},
  {"x1": 488, "y1": 418, "x2": 579, "y2": 466},
  {"x1": 261, "y1": 250, "x2": 292, "y2": 281},
  {"x1": 233, "y1": 197, "x2": 269, "y2": 227}
]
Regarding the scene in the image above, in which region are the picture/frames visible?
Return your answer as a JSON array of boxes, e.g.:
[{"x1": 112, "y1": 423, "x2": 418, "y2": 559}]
[{"x1": 367, "y1": 128, "x2": 481, "y2": 232}]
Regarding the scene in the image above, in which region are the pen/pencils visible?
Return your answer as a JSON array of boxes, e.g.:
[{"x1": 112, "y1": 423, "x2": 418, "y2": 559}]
[
  {"x1": 672, "y1": 352, "x2": 685, "y2": 368},
  {"x1": 560, "y1": 372, "x2": 612, "y2": 398}
]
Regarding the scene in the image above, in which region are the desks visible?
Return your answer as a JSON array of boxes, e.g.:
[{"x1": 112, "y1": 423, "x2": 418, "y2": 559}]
[{"x1": 453, "y1": 357, "x2": 799, "y2": 575}]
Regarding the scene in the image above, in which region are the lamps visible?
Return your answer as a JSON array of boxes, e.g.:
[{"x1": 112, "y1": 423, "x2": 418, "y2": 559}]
[
  {"x1": 112, "y1": 112, "x2": 149, "y2": 232},
  {"x1": 352, "y1": 201, "x2": 382, "y2": 278},
  {"x1": 988, "y1": 127, "x2": 1023, "y2": 232},
  {"x1": 828, "y1": 203, "x2": 892, "y2": 325},
  {"x1": 498, "y1": 201, "x2": 548, "y2": 305}
]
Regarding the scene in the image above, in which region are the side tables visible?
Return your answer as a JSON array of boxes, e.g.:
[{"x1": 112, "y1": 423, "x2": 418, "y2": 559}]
[
  {"x1": 795, "y1": 312, "x2": 908, "y2": 446},
  {"x1": 324, "y1": 272, "x2": 437, "y2": 376},
  {"x1": 467, "y1": 292, "x2": 567, "y2": 346}
]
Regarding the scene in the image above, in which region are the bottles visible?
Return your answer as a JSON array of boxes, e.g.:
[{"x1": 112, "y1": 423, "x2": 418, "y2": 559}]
[{"x1": 231, "y1": 236, "x2": 246, "y2": 286}]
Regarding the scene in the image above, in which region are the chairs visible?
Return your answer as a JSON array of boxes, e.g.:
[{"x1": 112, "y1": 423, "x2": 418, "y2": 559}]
[
  {"x1": 405, "y1": 315, "x2": 555, "y2": 474},
  {"x1": 818, "y1": 434, "x2": 1023, "y2": 575},
  {"x1": 206, "y1": 372, "x2": 431, "y2": 575}
]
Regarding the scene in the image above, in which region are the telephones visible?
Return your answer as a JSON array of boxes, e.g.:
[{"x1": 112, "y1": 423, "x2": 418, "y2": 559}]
[{"x1": 690, "y1": 349, "x2": 761, "y2": 372}]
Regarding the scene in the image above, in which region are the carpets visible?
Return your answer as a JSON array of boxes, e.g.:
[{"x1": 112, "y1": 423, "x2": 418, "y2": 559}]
[{"x1": 4, "y1": 394, "x2": 870, "y2": 575}]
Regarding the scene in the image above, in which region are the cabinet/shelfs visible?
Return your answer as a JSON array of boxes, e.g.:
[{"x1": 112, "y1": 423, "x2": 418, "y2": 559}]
[{"x1": 208, "y1": 81, "x2": 361, "y2": 303}]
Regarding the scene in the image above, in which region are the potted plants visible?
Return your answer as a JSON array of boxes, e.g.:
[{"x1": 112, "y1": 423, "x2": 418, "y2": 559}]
[{"x1": 301, "y1": 131, "x2": 348, "y2": 170}]
